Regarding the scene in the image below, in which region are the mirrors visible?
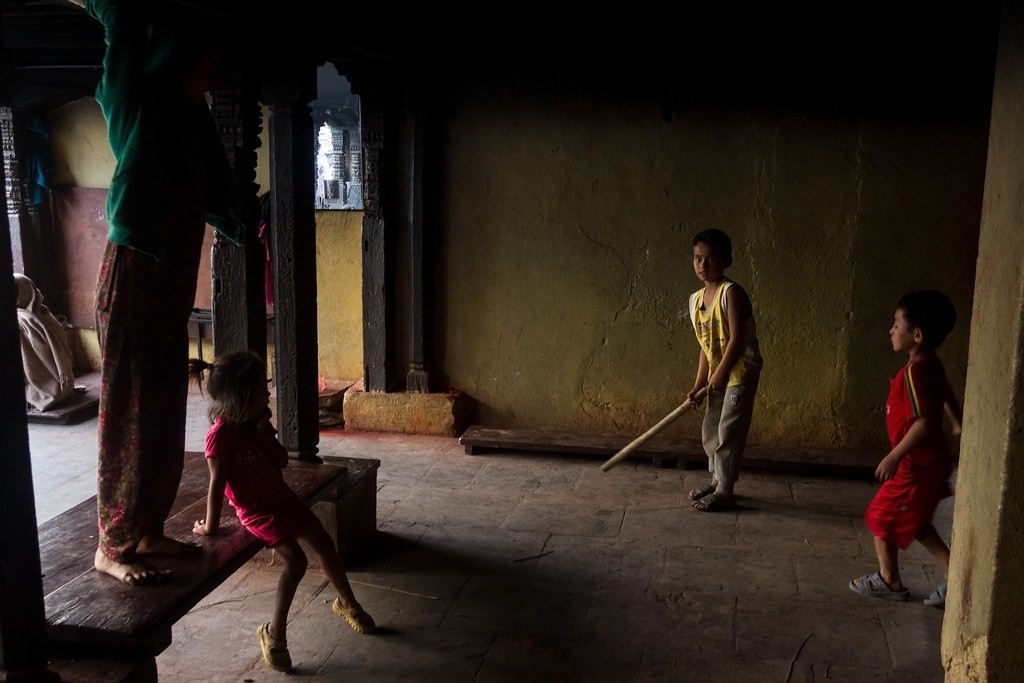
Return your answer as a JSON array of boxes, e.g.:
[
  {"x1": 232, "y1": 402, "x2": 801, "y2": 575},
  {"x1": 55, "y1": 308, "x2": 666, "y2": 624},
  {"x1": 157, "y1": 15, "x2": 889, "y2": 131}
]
[{"x1": 308, "y1": 60, "x2": 365, "y2": 209}]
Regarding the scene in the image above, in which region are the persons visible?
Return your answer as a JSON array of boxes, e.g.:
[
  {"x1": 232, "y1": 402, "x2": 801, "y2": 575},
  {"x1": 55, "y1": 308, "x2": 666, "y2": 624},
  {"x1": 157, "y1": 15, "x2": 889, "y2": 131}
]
[
  {"x1": 69, "y1": 0, "x2": 267, "y2": 586},
  {"x1": 192, "y1": 350, "x2": 374, "y2": 672},
  {"x1": 689, "y1": 228, "x2": 764, "y2": 513},
  {"x1": 848, "y1": 289, "x2": 963, "y2": 605}
]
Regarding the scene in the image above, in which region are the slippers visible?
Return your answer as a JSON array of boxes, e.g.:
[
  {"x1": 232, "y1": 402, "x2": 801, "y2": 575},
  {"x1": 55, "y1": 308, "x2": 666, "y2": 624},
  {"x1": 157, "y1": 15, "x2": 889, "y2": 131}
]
[
  {"x1": 688, "y1": 484, "x2": 716, "y2": 501},
  {"x1": 849, "y1": 570, "x2": 911, "y2": 601},
  {"x1": 692, "y1": 493, "x2": 738, "y2": 512},
  {"x1": 924, "y1": 579, "x2": 947, "y2": 606}
]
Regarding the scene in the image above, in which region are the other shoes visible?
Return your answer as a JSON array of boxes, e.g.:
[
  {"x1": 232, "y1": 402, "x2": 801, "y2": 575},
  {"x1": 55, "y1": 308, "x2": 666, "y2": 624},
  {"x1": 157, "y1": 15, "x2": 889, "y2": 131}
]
[
  {"x1": 256, "y1": 622, "x2": 292, "y2": 672},
  {"x1": 331, "y1": 595, "x2": 375, "y2": 634}
]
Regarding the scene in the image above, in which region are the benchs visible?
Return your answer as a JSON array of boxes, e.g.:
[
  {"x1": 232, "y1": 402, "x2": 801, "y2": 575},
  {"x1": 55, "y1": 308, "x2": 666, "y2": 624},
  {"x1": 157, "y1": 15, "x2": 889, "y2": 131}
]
[{"x1": 37, "y1": 449, "x2": 349, "y2": 683}]
